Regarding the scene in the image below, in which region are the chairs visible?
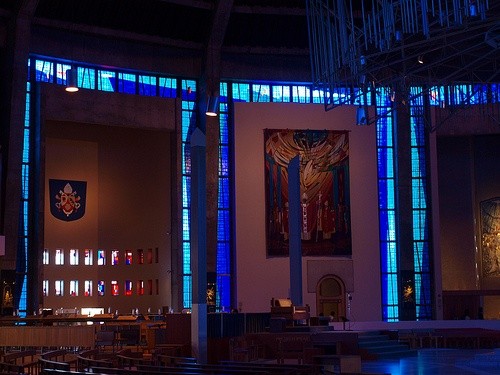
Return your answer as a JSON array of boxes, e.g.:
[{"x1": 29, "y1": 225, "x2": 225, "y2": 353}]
[{"x1": 270, "y1": 298, "x2": 310, "y2": 328}]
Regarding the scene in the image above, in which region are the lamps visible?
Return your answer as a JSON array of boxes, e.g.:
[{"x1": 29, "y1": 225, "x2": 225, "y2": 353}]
[
  {"x1": 64, "y1": 0, "x2": 79, "y2": 92},
  {"x1": 205, "y1": 0, "x2": 219, "y2": 117}
]
